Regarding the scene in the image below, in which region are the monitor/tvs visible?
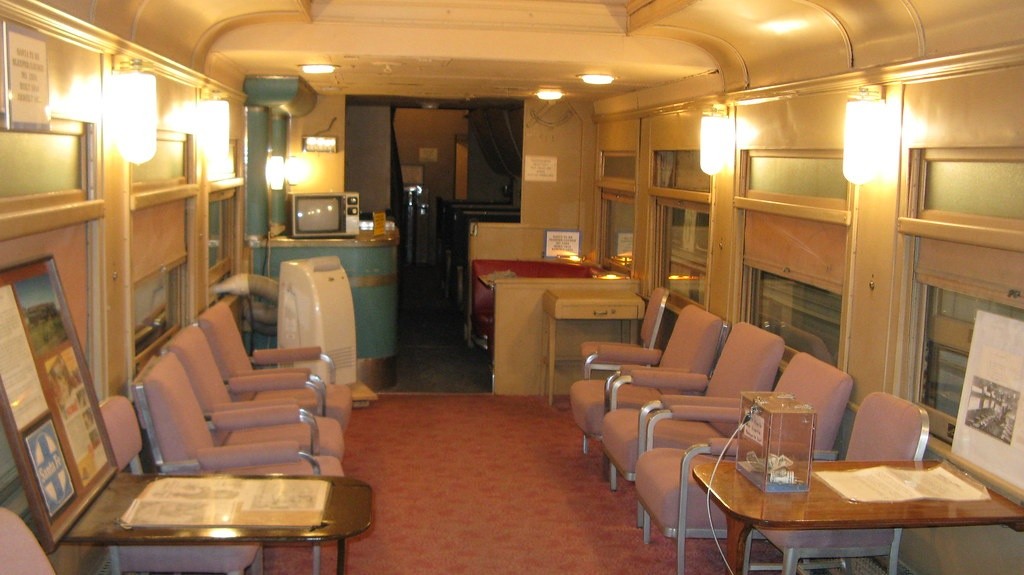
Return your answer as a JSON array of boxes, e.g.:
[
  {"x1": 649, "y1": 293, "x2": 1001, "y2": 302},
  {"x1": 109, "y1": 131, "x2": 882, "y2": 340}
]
[{"x1": 287, "y1": 192, "x2": 360, "y2": 238}]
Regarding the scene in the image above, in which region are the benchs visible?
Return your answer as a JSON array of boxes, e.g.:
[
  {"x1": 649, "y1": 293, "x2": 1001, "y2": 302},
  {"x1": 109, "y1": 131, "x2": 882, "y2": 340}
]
[
  {"x1": 473, "y1": 259, "x2": 591, "y2": 348},
  {"x1": 436, "y1": 198, "x2": 518, "y2": 315}
]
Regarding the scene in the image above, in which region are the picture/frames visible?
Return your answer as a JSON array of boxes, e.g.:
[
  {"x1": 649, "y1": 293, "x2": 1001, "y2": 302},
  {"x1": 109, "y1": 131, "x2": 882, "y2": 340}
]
[{"x1": 0, "y1": 254, "x2": 119, "y2": 555}]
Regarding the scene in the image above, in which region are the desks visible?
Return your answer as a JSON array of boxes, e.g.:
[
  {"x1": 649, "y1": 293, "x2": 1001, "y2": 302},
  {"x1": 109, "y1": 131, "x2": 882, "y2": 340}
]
[
  {"x1": 62, "y1": 472, "x2": 374, "y2": 575},
  {"x1": 541, "y1": 288, "x2": 645, "y2": 405},
  {"x1": 691, "y1": 459, "x2": 1024, "y2": 575},
  {"x1": 478, "y1": 274, "x2": 519, "y2": 290}
]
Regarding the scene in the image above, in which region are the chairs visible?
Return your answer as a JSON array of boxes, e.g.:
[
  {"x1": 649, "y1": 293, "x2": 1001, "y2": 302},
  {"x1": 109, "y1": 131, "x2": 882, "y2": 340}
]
[
  {"x1": 568, "y1": 286, "x2": 929, "y2": 574},
  {"x1": 0, "y1": 303, "x2": 353, "y2": 575}
]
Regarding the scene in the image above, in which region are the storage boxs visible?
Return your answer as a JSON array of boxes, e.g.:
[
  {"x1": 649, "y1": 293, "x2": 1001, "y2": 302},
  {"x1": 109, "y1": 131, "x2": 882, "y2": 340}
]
[{"x1": 735, "y1": 392, "x2": 817, "y2": 494}]
